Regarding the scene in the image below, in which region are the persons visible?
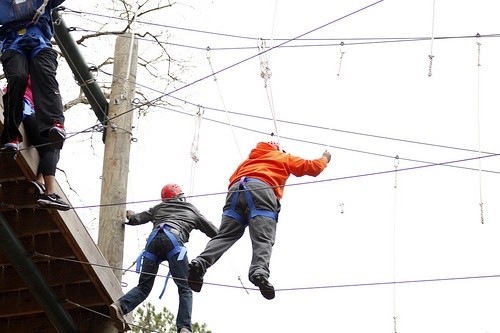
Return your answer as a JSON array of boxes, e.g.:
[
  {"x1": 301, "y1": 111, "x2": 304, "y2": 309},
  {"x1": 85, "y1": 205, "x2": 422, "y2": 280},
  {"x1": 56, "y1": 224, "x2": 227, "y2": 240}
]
[
  {"x1": 109, "y1": 184, "x2": 218, "y2": 333},
  {"x1": 0, "y1": 0, "x2": 72, "y2": 211},
  {"x1": 188, "y1": 142, "x2": 331, "y2": 300}
]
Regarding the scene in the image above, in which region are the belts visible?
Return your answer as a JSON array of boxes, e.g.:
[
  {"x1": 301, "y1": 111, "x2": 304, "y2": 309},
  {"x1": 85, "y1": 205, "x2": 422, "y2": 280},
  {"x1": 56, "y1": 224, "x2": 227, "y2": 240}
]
[{"x1": 166, "y1": 228, "x2": 182, "y2": 237}]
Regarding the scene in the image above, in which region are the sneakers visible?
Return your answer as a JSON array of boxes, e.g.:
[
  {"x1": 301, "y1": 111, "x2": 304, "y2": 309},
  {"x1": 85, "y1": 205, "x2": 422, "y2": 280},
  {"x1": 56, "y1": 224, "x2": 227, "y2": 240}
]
[
  {"x1": 5, "y1": 137, "x2": 19, "y2": 152},
  {"x1": 37, "y1": 193, "x2": 70, "y2": 212},
  {"x1": 29, "y1": 181, "x2": 46, "y2": 198},
  {"x1": 109, "y1": 302, "x2": 125, "y2": 331},
  {"x1": 179, "y1": 327, "x2": 193, "y2": 333},
  {"x1": 49, "y1": 123, "x2": 66, "y2": 141}
]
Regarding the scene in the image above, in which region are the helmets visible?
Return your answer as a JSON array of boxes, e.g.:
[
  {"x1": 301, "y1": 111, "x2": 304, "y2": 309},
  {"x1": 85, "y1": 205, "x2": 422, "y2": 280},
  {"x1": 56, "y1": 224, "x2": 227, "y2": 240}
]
[
  {"x1": 161, "y1": 184, "x2": 185, "y2": 202},
  {"x1": 268, "y1": 141, "x2": 286, "y2": 152}
]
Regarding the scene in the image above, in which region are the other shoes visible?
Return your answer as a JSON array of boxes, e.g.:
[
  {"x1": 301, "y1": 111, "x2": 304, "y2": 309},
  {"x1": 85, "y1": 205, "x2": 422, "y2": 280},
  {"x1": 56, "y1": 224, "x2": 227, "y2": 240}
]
[
  {"x1": 188, "y1": 259, "x2": 203, "y2": 292},
  {"x1": 253, "y1": 273, "x2": 275, "y2": 300}
]
